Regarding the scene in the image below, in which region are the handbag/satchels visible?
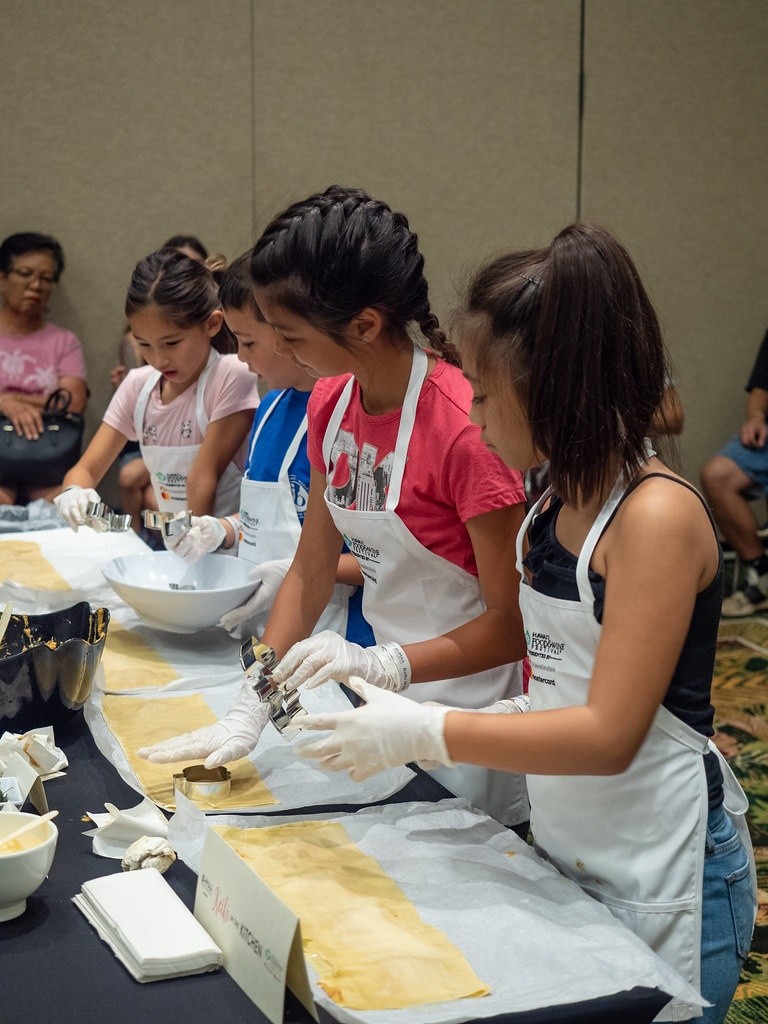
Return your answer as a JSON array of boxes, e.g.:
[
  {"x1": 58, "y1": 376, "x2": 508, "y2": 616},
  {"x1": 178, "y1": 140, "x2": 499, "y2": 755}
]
[{"x1": 0, "y1": 388, "x2": 85, "y2": 490}]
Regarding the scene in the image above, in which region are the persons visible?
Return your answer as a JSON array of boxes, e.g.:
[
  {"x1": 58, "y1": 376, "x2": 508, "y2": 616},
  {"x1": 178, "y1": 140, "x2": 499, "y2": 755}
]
[
  {"x1": 1, "y1": 231, "x2": 91, "y2": 507},
  {"x1": 702, "y1": 329, "x2": 768, "y2": 616},
  {"x1": 303, "y1": 222, "x2": 758, "y2": 1024},
  {"x1": 53, "y1": 236, "x2": 262, "y2": 556},
  {"x1": 162, "y1": 247, "x2": 375, "y2": 649},
  {"x1": 619, "y1": 377, "x2": 685, "y2": 436},
  {"x1": 137, "y1": 188, "x2": 530, "y2": 842}
]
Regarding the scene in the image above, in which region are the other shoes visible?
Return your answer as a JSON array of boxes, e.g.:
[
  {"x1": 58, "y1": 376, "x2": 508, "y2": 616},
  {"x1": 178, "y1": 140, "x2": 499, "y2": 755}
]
[{"x1": 720, "y1": 566, "x2": 768, "y2": 617}]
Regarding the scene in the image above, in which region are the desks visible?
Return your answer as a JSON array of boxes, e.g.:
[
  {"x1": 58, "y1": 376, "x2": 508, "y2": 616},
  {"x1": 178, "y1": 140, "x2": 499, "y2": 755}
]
[{"x1": 0, "y1": 515, "x2": 674, "y2": 1024}]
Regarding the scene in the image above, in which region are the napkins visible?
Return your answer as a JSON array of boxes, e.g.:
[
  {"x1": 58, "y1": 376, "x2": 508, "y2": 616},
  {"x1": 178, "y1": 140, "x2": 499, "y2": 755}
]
[
  {"x1": 82, "y1": 796, "x2": 170, "y2": 862},
  {"x1": 71, "y1": 867, "x2": 225, "y2": 983}
]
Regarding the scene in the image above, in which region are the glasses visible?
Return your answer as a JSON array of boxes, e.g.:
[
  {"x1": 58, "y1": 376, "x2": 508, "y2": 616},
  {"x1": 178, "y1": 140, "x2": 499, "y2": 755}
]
[{"x1": 4, "y1": 266, "x2": 57, "y2": 287}]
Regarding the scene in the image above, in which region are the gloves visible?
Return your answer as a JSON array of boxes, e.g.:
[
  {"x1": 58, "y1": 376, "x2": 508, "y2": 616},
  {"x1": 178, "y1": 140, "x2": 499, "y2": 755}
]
[
  {"x1": 413, "y1": 699, "x2": 523, "y2": 772},
  {"x1": 290, "y1": 675, "x2": 463, "y2": 783},
  {"x1": 271, "y1": 629, "x2": 411, "y2": 693},
  {"x1": 163, "y1": 516, "x2": 227, "y2": 566},
  {"x1": 136, "y1": 679, "x2": 271, "y2": 770},
  {"x1": 54, "y1": 485, "x2": 107, "y2": 533},
  {"x1": 220, "y1": 559, "x2": 296, "y2": 631}
]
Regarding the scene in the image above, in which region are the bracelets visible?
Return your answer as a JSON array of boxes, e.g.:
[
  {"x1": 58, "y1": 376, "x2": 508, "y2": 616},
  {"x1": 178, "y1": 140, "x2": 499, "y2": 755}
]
[{"x1": 222, "y1": 516, "x2": 240, "y2": 551}]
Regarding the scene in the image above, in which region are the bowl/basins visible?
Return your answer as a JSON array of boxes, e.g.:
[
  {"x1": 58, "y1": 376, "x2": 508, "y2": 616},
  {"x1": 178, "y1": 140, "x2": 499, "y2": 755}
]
[
  {"x1": 0, "y1": 810, "x2": 59, "y2": 922},
  {"x1": 102, "y1": 550, "x2": 263, "y2": 631},
  {"x1": 0, "y1": 601, "x2": 110, "y2": 733}
]
